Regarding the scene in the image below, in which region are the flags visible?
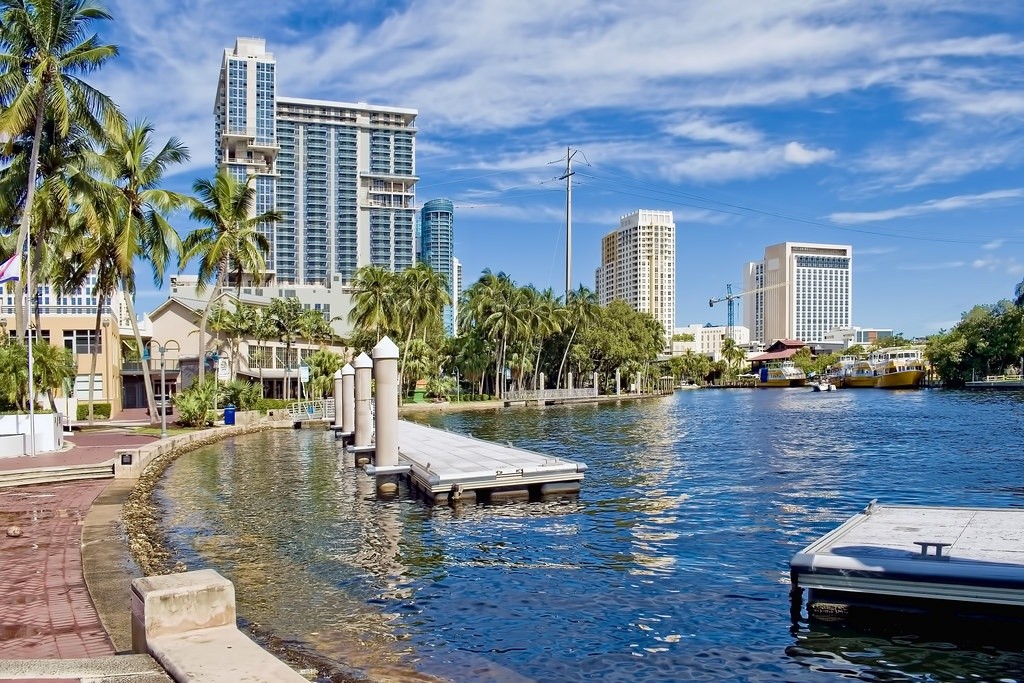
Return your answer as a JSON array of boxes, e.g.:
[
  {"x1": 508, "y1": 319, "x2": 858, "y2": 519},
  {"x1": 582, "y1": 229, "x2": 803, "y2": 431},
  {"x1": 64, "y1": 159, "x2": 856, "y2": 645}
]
[{"x1": 0, "y1": 251, "x2": 21, "y2": 284}]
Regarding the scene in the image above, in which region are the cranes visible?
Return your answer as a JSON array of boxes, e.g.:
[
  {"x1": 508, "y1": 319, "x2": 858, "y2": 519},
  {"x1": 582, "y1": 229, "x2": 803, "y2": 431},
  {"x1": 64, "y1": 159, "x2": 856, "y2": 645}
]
[{"x1": 710, "y1": 281, "x2": 792, "y2": 341}]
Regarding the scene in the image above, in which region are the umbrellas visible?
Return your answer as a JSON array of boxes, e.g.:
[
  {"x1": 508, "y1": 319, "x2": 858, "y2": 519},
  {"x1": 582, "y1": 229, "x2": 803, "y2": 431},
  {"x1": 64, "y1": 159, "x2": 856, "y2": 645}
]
[{"x1": 736, "y1": 373, "x2": 760, "y2": 380}]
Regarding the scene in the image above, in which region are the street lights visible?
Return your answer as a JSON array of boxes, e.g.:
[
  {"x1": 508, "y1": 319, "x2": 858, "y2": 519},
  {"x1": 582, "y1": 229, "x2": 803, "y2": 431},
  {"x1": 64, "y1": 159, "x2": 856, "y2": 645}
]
[
  {"x1": 204, "y1": 350, "x2": 231, "y2": 424},
  {"x1": 286, "y1": 366, "x2": 294, "y2": 401},
  {"x1": 451, "y1": 366, "x2": 460, "y2": 402},
  {"x1": 142, "y1": 339, "x2": 184, "y2": 440}
]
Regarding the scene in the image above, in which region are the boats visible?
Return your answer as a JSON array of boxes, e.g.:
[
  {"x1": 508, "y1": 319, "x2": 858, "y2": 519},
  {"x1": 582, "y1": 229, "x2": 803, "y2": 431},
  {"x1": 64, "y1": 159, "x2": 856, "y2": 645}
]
[
  {"x1": 808, "y1": 375, "x2": 837, "y2": 392},
  {"x1": 830, "y1": 361, "x2": 875, "y2": 387},
  {"x1": 675, "y1": 381, "x2": 701, "y2": 389},
  {"x1": 873, "y1": 360, "x2": 925, "y2": 389},
  {"x1": 755, "y1": 368, "x2": 806, "y2": 387}
]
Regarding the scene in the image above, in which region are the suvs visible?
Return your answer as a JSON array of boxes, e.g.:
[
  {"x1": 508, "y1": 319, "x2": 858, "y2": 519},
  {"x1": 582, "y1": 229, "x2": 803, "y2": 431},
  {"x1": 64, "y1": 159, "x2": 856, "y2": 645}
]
[{"x1": 147, "y1": 393, "x2": 173, "y2": 414}]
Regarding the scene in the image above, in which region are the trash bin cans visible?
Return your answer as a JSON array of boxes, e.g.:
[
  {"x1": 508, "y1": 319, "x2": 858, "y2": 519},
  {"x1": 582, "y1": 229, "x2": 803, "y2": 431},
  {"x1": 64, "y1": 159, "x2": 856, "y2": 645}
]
[{"x1": 225, "y1": 406, "x2": 235, "y2": 425}]
